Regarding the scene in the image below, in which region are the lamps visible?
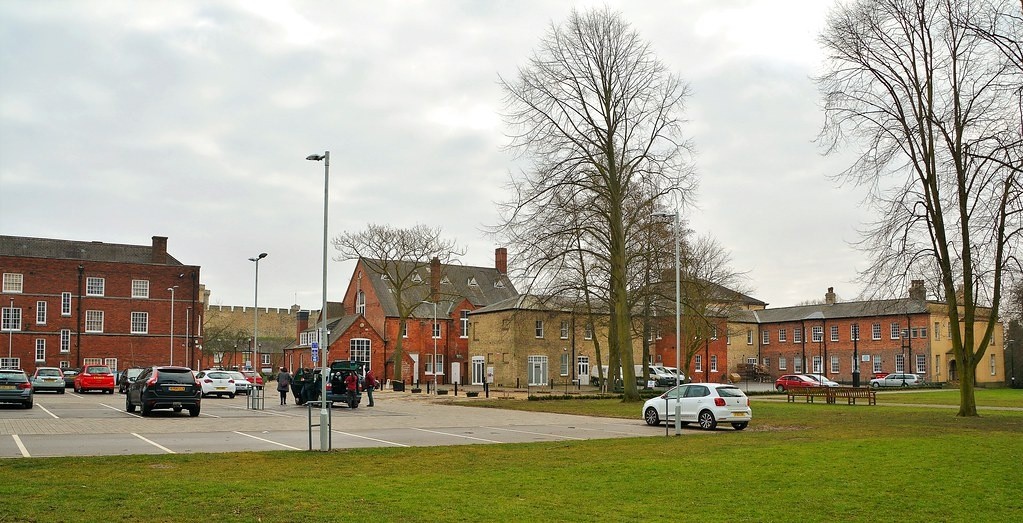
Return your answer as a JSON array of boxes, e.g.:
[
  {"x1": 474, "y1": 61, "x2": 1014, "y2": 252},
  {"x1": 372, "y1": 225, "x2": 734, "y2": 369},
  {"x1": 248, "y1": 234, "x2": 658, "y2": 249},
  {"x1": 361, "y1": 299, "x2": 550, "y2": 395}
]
[
  {"x1": 188, "y1": 338, "x2": 199, "y2": 347},
  {"x1": 176, "y1": 272, "x2": 184, "y2": 279}
]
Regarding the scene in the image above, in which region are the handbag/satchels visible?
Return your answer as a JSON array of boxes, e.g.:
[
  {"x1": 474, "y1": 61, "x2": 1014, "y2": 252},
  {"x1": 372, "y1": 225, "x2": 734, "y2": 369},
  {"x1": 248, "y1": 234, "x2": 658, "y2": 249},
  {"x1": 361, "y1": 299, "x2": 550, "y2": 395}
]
[{"x1": 281, "y1": 380, "x2": 288, "y2": 387}]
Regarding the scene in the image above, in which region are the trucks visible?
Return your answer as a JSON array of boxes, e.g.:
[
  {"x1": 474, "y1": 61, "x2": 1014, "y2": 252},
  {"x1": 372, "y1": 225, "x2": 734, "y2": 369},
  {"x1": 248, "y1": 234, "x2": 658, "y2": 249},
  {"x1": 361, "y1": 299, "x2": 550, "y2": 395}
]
[{"x1": 591, "y1": 365, "x2": 691, "y2": 386}]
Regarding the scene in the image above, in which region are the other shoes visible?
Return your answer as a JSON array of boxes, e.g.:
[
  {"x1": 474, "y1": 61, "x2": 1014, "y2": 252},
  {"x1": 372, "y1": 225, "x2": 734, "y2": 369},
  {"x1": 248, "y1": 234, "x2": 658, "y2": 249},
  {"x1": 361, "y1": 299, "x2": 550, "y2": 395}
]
[
  {"x1": 367, "y1": 404, "x2": 373, "y2": 407},
  {"x1": 284, "y1": 403, "x2": 286, "y2": 404},
  {"x1": 281, "y1": 401, "x2": 283, "y2": 405}
]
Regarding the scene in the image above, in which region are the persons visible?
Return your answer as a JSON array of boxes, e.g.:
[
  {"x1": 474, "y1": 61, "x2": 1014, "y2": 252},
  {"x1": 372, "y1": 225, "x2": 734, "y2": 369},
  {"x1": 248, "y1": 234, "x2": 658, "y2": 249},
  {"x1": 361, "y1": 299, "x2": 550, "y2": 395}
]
[
  {"x1": 276, "y1": 367, "x2": 292, "y2": 405},
  {"x1": 365, "y1": 367, "x2": 379, "y2": 407},
  {"x1": 345, "y1": 371, "x2": 358, "y2": 407},
  {"x1": 314, "y1": 374, "x2": 322, "y2": 406},
  {"x1": 300, "y1": 367, "x2": 313, "y2": 407}
]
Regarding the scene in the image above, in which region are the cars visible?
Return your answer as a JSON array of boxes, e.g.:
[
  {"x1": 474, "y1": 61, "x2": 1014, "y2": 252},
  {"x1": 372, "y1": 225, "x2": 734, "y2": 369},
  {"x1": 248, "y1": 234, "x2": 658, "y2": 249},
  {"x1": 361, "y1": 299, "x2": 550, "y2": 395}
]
[
  {"x1": 192, "y1": 370, "x2": 236, "y2": 398},
  {"x1": 775, "y1": 374, "x2": 839, "y2": 392},
  {"x1": 118, "y1": 367, "x2": 146, "y2": 393},
  {"x1": 63, "y1": 368, "x2": 77, "y2": 388},
  {"x1": 642, "y1": 383, "x2": 753, "y2": 431}
]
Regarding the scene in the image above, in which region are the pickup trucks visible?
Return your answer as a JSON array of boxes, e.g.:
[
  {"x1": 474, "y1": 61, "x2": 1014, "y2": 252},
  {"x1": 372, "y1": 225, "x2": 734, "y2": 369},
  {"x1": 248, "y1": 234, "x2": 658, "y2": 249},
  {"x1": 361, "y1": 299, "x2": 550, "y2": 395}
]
[{"x1": 869, "y1": 373, "x2": 920, "y2": 387}]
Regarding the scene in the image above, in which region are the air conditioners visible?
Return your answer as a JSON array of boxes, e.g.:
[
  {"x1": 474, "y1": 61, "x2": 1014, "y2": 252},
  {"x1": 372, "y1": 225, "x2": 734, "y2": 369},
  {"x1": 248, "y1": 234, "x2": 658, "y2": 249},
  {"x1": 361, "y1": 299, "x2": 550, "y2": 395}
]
[{"x1": 25, "y1": 323, "x2": 32, "y2": 330}]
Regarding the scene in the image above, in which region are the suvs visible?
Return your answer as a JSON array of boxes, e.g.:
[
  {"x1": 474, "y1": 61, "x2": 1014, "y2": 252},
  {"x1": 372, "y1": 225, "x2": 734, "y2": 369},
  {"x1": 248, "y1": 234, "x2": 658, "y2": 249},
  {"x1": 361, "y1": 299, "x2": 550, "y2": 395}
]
[
  {"x1": 0, "y1": 367, "x2": 66, "y2": 408},
  {"x1": 126, "y1": 366, "x2": 203, "y2": 417},
  {"x1": 291, "y1": 360, "x2": 363, "y2": 408},
  {"x1": 226, "y1": 371, "x2": 261, "y2": 396},
  {"x1": 74, "y1": 364, "x2": 115, "y2": 394}
]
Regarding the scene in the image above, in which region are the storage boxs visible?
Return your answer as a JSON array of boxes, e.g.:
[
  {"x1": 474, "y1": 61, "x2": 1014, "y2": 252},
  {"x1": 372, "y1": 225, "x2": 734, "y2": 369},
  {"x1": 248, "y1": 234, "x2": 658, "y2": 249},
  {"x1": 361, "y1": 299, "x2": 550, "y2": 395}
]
[{"x1": 731, "y1": 373, "x2": 741, "y2": 381}]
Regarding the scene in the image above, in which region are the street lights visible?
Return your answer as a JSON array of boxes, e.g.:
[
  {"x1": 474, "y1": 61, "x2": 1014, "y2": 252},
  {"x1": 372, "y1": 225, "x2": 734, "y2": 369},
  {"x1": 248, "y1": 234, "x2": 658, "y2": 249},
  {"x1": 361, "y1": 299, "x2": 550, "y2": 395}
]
[
  {"x1": 167, "y1": 285, "x2": 179, "y2": 366},
  {"x1": 422, "y1": 300, "x2": 437, "y2": 395},
  {"x1": 817, "y1": 332, "x2": 825, "y2": 386},
  {"x1": 650, "y1": 211, "x2": 682, "y2": 437},
  {"x1": 306, "y1": 151, "x2": 329, "y2": 451},
  {"x1": 248, "y1": 252, "x2": 268, "y2": 409},
  {"x1": 900, "y1": 332, "x2": 906, "y2": 387},
  {"x1": 1008, "y1": 338, "x2": 1016, "y2": 387}
]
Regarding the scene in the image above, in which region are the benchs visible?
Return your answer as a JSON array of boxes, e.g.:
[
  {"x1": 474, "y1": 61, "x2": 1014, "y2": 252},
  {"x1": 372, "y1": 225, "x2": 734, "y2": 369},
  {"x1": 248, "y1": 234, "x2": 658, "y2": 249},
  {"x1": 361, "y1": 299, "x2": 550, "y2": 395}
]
[{"x1": 787, "y1": 386, "x2": 876, "y2": 405}]
